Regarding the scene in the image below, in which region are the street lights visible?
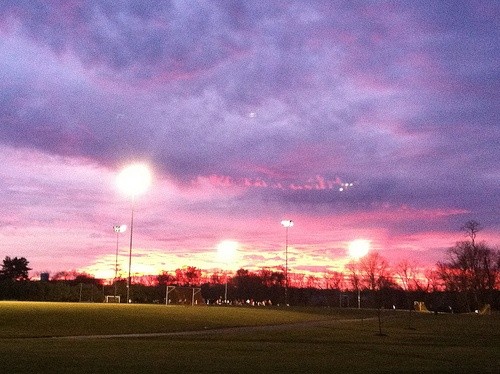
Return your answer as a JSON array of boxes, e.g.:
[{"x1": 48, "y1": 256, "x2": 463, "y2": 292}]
[
  {"x1": 112, "y1": 224, "x2": 128, "y2": 296},
  {"x1": 280, "y1": 218, "x2": 294, "y2": 306}
]
